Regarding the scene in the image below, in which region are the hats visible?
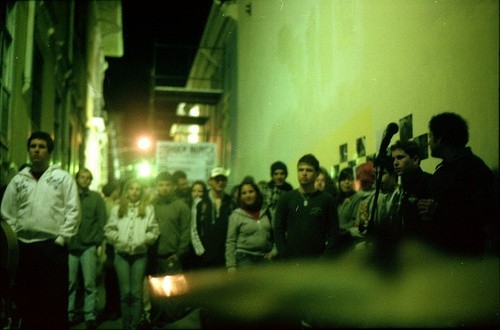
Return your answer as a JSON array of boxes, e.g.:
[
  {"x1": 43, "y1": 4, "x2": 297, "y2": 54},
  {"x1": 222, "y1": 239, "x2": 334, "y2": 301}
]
[
  {"x1": 356, "y1": 163, "x2": 374, "y2": 180},
  {"x1": 211, "y1": 167, "x2": 229, "y2": 177},
  {"x1": 102, "y1": 182, "x2": 117, "y2": 194}
]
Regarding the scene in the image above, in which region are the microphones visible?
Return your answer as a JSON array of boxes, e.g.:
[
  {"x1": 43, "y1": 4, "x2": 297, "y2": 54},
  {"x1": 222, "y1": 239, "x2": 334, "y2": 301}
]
[{"x1": 378, "y1": 123, "x2": 399, "y2": 169}]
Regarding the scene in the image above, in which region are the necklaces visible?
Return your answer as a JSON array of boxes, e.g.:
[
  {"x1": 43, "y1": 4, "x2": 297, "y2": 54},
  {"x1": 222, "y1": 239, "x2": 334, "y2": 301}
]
[{"x1": 299, "y1": 188, "x2": 314, "y2": 208}]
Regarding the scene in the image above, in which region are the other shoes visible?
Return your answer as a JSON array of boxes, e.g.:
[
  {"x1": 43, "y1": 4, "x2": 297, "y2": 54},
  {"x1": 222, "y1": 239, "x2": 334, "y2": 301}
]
[
  {"x1": 72, "y1": 315, "x2": 83, "y2": 323},
  {"x1": 111, "y1": 312, "x2": 116, "y2": 320},
  {"x1": 87, "y1": 320, "x2": 96, "y2": 330}
]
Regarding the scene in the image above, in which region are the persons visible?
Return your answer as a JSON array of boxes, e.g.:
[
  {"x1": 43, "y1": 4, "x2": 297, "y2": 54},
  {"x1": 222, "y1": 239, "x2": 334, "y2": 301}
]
[
  {"x1": 0, "y1": 131, "x2": 82, "y2": 330},
  {"x1": 67, "y1": 111, "x2": 500, "y2": 330}
]
